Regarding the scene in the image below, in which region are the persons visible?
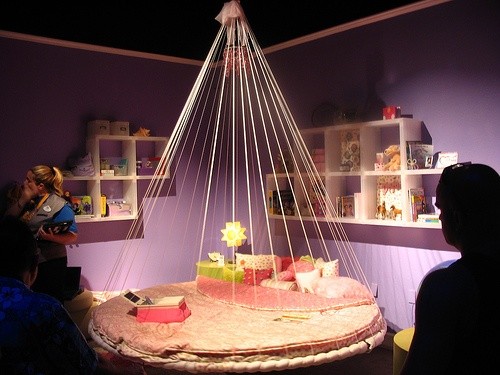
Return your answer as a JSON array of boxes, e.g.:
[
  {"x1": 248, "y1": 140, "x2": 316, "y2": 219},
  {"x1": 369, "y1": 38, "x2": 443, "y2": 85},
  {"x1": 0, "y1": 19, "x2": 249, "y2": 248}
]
[
  {"x1": 399, "y1": 161, "x2": 500, "y2": 375},
  {"x1": 0, "y1": 166, "x2": 98, "y2": 375}
]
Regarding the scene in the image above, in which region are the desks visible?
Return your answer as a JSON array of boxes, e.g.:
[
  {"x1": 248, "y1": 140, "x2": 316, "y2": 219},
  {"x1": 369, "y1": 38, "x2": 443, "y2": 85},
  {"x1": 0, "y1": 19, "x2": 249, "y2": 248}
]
[
  {"x1": 195, "y1": 259, "x2": 234, "y2": 280},
  {"x1": 392, "y1": 327, "x2": 415, "y2": 375},
  {"x1": 311, "y1": 149, "x2": 325, "y2": 171}
]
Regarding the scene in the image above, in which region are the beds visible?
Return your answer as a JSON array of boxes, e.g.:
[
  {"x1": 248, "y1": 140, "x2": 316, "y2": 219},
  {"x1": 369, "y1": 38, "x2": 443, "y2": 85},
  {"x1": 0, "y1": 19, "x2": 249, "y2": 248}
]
[{"x1": 88, "y1": 0, "x2": 386, "y2": 375}]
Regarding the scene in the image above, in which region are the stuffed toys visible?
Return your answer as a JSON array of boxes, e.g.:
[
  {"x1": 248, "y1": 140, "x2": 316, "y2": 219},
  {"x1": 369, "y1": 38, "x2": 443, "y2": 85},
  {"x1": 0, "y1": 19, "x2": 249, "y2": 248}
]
[{"x1": 383, "y1": 144, "x2": 400, "y2": 172}]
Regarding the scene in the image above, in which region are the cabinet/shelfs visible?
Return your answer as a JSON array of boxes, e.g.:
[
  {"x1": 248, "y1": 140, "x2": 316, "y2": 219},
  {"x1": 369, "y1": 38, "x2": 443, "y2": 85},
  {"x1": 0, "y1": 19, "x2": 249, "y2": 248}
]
[
  {"x1": 63, "y1": 134, "x2": 170, "y2": 224},
  {"x1": 266, "y1": 118, "x2": 447, "y2": 229}
]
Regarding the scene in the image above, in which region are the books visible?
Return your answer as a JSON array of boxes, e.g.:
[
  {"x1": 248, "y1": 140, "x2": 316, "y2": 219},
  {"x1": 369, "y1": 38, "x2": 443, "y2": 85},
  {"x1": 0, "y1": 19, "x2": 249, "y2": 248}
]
[{"x1": 269, "y1": 184, "x2": 441, "y2": 224}]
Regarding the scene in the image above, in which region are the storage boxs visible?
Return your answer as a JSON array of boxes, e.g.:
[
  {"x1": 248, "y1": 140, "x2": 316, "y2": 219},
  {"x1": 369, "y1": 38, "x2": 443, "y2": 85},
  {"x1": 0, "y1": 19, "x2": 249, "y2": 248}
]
[
  {"x1": 111, "y1": 164, "x2": 127, "y2": 175},
  {"x1": 136, "y1": 303, "x2": 191, "y2": 323},
  {"x1": 88, "y1": 120, "x2": 129, "y2": 136}
]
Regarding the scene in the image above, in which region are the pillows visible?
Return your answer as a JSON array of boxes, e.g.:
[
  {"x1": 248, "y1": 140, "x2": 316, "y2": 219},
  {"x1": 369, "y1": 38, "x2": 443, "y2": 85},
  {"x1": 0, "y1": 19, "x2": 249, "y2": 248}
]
[{"x1": 234, "y1": 252, "x2": 372, "y2": 299}]
